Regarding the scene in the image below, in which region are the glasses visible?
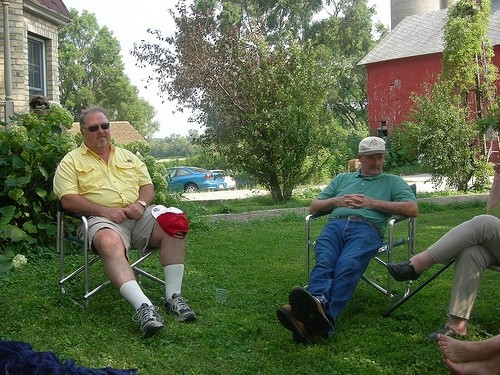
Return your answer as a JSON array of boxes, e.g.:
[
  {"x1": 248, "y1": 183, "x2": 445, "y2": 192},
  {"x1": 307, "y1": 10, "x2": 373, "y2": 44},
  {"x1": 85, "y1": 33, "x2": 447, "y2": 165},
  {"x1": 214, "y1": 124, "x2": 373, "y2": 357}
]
[{"x1": 87, "y1": 122, "x2": 109, "y2": 132}]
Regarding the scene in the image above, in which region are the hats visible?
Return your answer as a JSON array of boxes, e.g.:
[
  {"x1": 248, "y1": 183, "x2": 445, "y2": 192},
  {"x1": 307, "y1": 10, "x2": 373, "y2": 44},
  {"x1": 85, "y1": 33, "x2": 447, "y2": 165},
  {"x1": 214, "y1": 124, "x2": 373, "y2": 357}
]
[
  {"x1": 150, "y1": 205, "x2": 188, "y2": 239},
  {"x1": 358, "y1": 136, "x2": 386, "y2": 155}
]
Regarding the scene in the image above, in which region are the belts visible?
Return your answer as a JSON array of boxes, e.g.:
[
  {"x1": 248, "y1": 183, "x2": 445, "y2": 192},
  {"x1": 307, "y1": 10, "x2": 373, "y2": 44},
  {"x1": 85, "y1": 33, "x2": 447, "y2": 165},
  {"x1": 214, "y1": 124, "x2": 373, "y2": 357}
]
[{"x1": 336, "y1": 215, "x2": 367, "y2": 222}]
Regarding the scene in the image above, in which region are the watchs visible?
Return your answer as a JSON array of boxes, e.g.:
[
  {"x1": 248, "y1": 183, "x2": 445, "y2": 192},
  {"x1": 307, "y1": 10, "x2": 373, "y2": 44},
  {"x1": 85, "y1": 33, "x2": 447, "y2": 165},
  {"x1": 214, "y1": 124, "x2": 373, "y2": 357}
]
[{"x1": 136, "y1": 200, "x2": 146, "y2": 210}]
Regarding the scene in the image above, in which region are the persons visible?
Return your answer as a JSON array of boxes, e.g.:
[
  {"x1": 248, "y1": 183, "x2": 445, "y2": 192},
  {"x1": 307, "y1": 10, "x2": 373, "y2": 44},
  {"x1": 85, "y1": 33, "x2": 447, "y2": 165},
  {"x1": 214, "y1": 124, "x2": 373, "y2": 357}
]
[
  {"x1": 387, "y1": 164, "x2": 500, "y2": 344},
  {"x1": 436, "y1": 333, "x2": 500, "y2": 375},
  {"x1": 276, "y1": 137, "x2": 419, "y2": 344},
  {"x1": 53, "y1": 108, "x2": 197, "y2": 332},
  {"x1": 30, "y1": 96, "x2": 72, "y2": 133}
]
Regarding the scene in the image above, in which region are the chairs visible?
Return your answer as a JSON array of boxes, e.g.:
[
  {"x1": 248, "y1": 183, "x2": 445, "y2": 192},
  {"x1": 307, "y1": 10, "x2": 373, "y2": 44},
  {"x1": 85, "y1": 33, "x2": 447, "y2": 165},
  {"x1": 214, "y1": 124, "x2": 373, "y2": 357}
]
[
  {"x1": 305, "y1": 184, "x2": 417, "y2": 299},
  {"x1": 56, "y1": 200, "x2": 165, "y2": 308}
]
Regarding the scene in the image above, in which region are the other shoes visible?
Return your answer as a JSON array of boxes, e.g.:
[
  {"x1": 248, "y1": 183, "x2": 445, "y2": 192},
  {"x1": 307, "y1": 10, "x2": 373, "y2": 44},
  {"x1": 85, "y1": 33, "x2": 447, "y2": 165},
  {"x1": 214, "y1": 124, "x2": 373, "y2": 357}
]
[
  {"x1": 276, "y1": 304, "x2": 310, "y2": 344},
  {"x1": 386, "y1": 258, "x2": 421, "y2": 281},
  {"x1": 289, "y1": 286, "x2": 331, "y2": 337},
  {"x1": 423, "y1": 326, "x2": 467, "y2": 343}
]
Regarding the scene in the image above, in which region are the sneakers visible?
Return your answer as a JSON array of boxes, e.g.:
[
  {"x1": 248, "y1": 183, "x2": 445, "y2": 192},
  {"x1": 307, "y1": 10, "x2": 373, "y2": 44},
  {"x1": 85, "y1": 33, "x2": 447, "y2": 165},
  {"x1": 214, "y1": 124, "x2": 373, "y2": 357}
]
[
  {"x1": 132, "y1": 303, "x2": 165, "y2": 338},
  {"x1": 161, "y1": 293, "x2": 196, "y2": 323}
]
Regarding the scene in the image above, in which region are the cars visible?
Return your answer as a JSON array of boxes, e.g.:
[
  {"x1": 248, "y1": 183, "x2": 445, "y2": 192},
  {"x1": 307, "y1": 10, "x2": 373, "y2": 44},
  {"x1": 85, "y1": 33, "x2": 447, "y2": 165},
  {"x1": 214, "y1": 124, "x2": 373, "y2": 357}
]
[{"x1": 167, "y1": 166, "x2": 236, "y2": 193}]
